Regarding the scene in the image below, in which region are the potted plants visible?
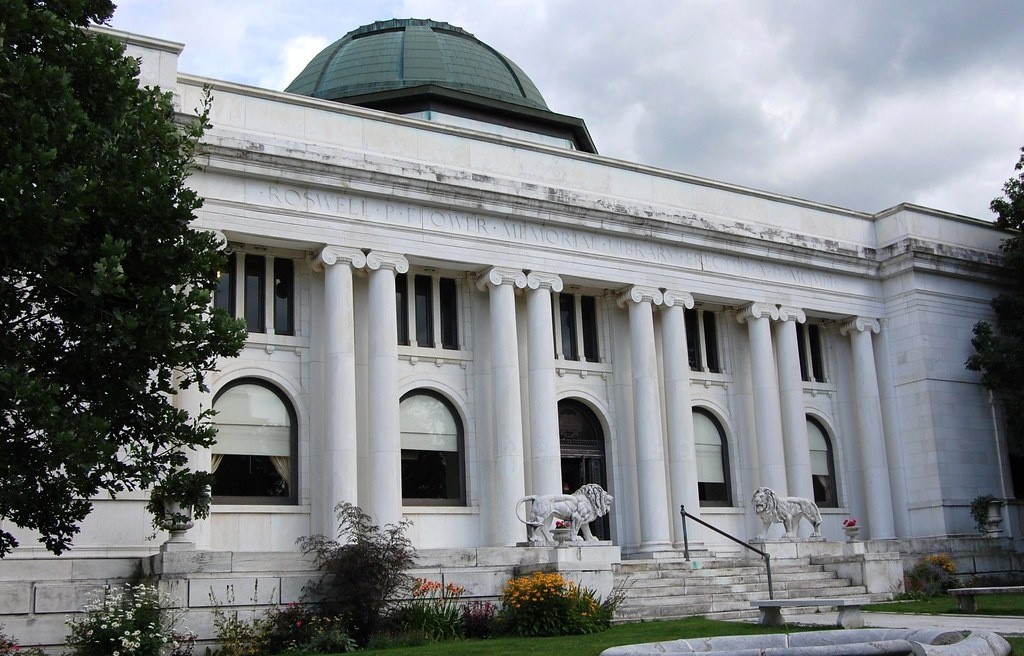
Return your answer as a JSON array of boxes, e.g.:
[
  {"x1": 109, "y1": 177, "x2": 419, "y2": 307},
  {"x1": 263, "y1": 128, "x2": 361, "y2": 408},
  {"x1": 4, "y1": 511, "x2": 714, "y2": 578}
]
[
  {"x1": 972, "y1": 492, "x2": 1008, "y2": 533},
  {"x1": 143, "y1": 468, "x2": 215, "y2": 541}
]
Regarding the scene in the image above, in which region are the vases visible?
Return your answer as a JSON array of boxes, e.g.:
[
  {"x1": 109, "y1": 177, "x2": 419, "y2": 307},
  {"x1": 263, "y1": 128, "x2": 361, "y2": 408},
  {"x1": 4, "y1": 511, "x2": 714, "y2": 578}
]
[
  {"x1": 550, "y1": 529, "x2": 572, "y2": 548},
  {"x1": 842, "y1": 526, "x2": 863, "y2": 543}
]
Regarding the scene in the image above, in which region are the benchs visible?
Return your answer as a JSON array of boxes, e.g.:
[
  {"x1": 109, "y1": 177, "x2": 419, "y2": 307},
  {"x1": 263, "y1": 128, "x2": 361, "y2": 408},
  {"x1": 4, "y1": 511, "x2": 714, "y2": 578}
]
[
  {"x1": 750, "y1": 596, "x2": 871, "y2": 629},
  {"x1": 948, "y1": 586, "x2": 1024, "y2": 613}
]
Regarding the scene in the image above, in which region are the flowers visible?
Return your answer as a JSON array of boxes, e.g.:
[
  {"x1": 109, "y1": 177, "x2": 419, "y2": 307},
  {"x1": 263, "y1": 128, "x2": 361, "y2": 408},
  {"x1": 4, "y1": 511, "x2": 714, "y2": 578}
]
[
  {"x1": 843, "y1": 518, "x2": 857, "y2": 527},
  {"x1": 556, "y1": 521, "x2": 571, "y2": 529}
]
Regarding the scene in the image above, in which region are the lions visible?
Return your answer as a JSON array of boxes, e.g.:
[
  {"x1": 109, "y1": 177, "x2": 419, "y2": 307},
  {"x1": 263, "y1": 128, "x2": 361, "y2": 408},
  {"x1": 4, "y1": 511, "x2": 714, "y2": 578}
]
[
  {"x1": 515, "y1": 484, "x2": 614, "y2": 541},
  {"x1": 752, "y1": 487, "x2": 823, "y2": 539}
]
